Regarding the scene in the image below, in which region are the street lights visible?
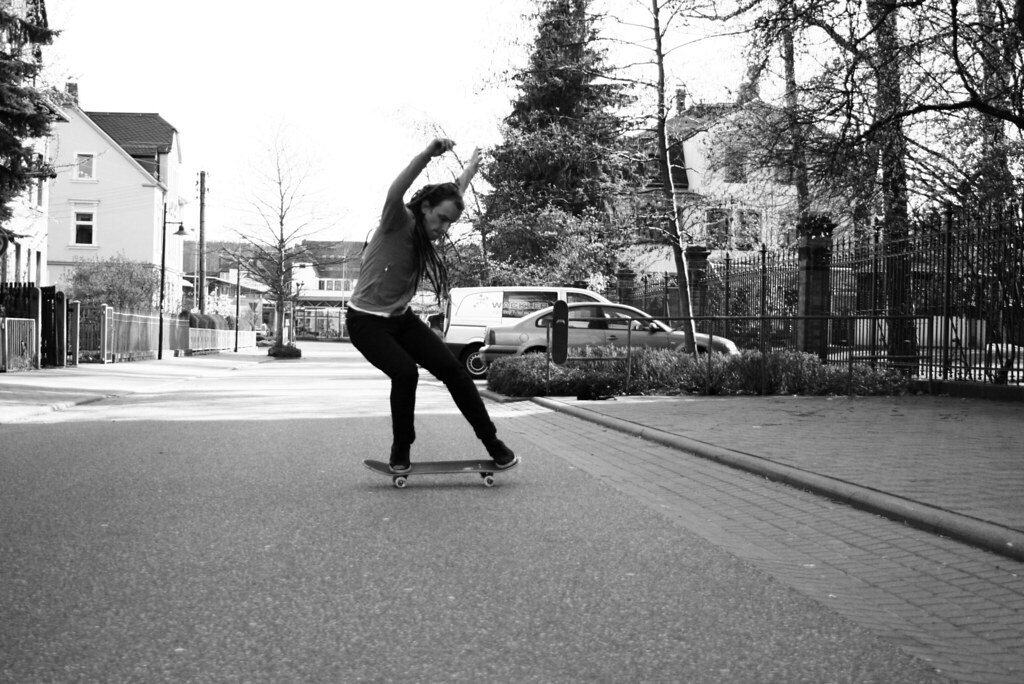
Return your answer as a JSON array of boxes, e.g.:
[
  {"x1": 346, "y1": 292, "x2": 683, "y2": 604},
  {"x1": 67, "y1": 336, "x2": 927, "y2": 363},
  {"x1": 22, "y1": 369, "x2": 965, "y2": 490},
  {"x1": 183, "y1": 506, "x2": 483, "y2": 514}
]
[
  {"x1": 157, "y1": 222, "x2": 190, "y2": 359},
  {"x1": 235, "y1": 270, "x2": 254, "y2": 353}
]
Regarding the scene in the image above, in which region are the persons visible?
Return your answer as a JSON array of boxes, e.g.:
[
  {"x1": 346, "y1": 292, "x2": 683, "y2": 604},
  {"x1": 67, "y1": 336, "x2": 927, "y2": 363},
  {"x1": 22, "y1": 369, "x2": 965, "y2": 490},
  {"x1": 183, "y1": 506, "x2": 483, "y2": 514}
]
[{"x1": 345, "y1": 137, "x2": 519, "y2": 476}]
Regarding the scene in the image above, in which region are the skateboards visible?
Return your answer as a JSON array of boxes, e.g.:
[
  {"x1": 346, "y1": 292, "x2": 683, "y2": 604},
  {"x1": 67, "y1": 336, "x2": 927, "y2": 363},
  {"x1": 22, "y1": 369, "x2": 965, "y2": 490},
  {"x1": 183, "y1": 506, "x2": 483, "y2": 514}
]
[{"x1": 362, "y1": 456, "x2": 522, "y2": 488}]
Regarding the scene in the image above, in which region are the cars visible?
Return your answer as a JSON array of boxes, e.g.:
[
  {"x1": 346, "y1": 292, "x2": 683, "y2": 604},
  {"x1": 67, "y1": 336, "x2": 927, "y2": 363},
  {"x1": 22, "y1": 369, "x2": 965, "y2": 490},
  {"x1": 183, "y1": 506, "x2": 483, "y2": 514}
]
[
  {"x1": 478, "y1": 301, "x2": 743, "y2": 369},
  {"x1": 256, "y1": 328, "x2": 267, "y2": 340}
]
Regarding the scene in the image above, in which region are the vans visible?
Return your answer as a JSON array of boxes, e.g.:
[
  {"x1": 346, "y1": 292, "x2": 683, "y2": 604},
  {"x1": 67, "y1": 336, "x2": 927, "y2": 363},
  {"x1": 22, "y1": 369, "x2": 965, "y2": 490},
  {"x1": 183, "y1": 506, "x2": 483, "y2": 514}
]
[
  {"x1": 424, "y1": 314, "x2": 443, "y2": 339},
  {"x1": 442, "y1": 286, "x2": 643, "y2": 379}
]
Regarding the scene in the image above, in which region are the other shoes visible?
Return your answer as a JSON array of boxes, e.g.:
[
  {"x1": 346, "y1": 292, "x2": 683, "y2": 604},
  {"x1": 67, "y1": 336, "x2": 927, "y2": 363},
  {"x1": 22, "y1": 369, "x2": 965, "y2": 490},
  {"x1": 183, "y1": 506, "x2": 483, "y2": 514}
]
[
  {"x1": 487, "y1": 440, "x2": 516, "y2": 468},
  {"x1": 389, "y1": 443, "x2": 412, "y2": 472}
]
